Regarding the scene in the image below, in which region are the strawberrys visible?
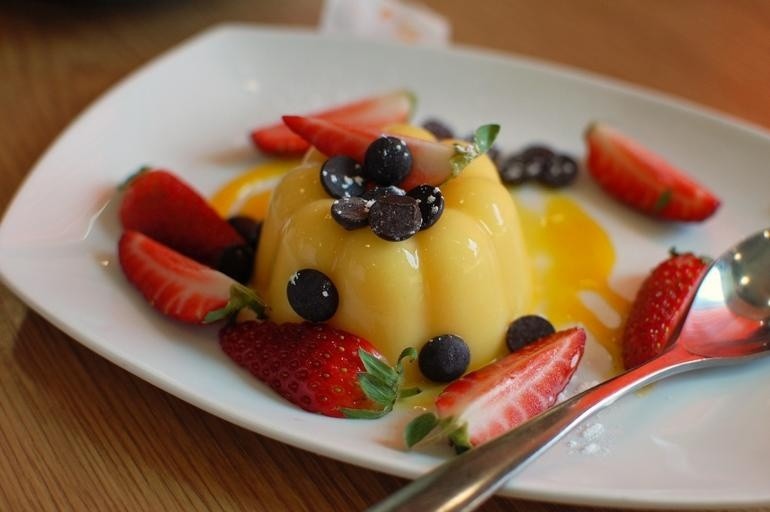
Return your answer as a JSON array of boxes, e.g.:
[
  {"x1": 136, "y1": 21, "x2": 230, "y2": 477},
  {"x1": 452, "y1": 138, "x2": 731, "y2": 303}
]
[
  {"x1": 404, "y1": 325, "x2": 586, "y2": 455},
  {"x1": 251, "y1": 89, "x2": 421, "y2": 156},
  {"x1": 121, "y1": 167, "x2": 248, "y2": 272},
  {"x1": 622, "y1": 244, "x2": 714, "y2": 370},
  {"x1": 219, "y1": 319, "x2": 423, "y2": 420},
  {"x1": 586, "y1": 122, "x2": 720, "y2": 222},
  {"x1": 280, "y1": 115, "x2": 501, "y2": 188},
  {"x1": 119, "y1": 227, "x2": 270, "y2": 326}
]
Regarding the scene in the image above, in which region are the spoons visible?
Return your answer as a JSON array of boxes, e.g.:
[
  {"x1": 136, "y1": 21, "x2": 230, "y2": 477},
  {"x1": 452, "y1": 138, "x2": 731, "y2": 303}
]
[{"x1": 366, "y1": 224, "x2": 769, "y2": 512}]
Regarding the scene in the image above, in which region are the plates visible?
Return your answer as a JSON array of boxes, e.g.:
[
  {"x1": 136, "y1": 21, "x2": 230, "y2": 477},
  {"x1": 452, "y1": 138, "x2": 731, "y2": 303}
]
[{"x1": 0, "y1": 23, "x2": 770, "y2": 511}]
